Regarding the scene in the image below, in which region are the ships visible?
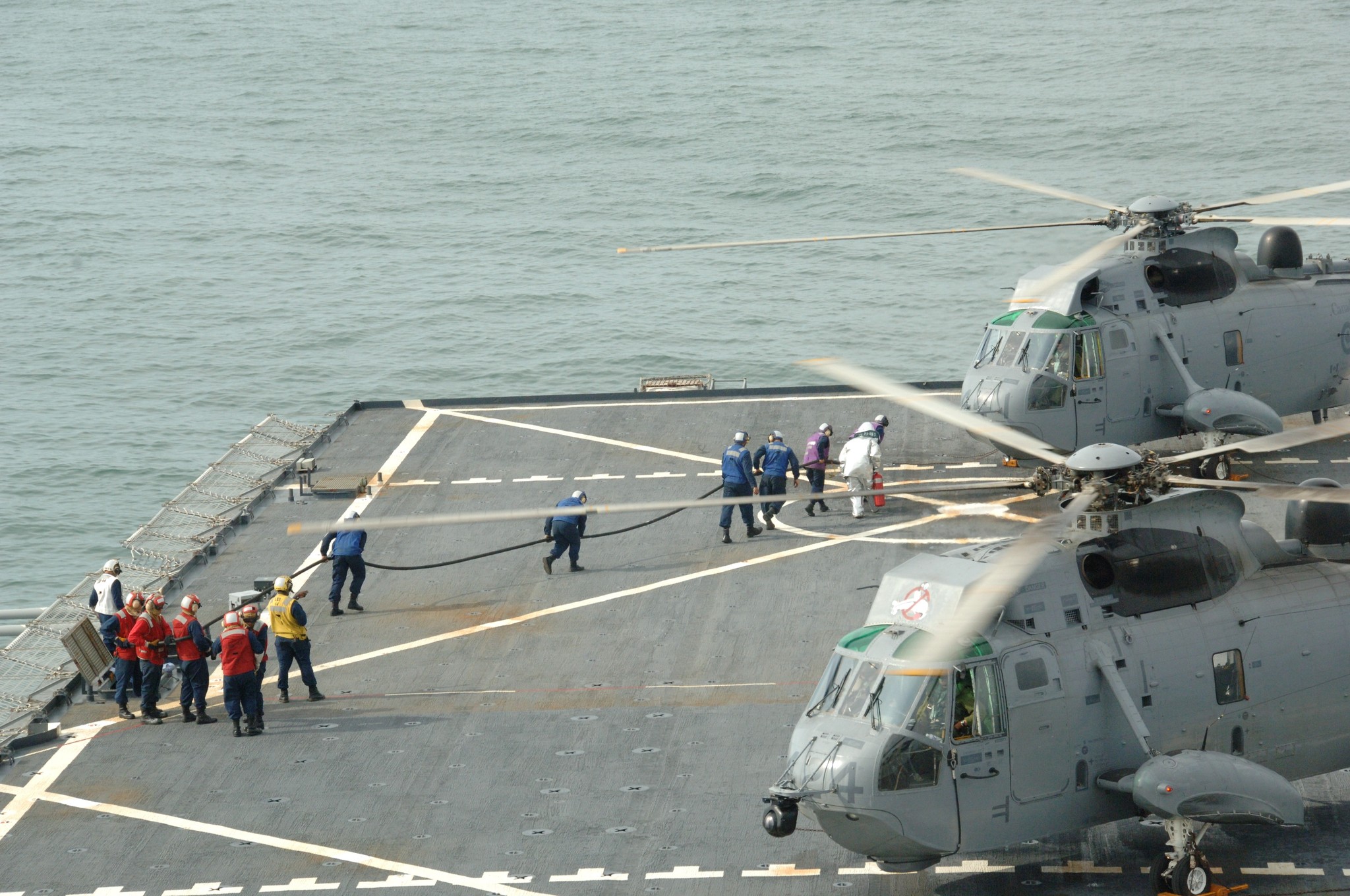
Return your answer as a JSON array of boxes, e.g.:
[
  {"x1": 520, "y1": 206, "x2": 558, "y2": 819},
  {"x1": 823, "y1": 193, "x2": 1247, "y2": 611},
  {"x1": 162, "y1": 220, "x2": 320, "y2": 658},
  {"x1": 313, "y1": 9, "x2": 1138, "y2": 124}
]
[{"x1": 2, "y1": 371, "x2": 1347, "y2": 896}]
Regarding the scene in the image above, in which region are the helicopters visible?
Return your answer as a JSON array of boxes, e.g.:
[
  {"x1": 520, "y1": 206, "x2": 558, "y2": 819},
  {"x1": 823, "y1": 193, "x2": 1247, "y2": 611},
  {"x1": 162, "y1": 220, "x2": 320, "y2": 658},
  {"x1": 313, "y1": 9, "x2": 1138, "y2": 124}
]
[
  {"x1": 612, "y1": 167, "x2": 1350, "y2": 486},
  {"x1": 280, "y1": 361, "x2": 1350, "y2": 896}
]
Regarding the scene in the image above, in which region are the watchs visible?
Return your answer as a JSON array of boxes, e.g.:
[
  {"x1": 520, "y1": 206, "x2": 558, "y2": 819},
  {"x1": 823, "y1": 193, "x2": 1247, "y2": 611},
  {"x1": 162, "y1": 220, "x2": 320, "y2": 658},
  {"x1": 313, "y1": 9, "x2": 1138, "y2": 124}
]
[{"x1": 960, "y1": 720, "x2": 966, "y2": 727}]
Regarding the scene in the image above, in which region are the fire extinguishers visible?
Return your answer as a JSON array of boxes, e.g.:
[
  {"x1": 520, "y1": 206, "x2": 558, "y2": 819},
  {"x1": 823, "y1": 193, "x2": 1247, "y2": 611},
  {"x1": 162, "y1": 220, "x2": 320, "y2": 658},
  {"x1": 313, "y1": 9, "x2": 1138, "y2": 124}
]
[{"x1": 872, "y1": 472, "x2": 885, "y2": 507}]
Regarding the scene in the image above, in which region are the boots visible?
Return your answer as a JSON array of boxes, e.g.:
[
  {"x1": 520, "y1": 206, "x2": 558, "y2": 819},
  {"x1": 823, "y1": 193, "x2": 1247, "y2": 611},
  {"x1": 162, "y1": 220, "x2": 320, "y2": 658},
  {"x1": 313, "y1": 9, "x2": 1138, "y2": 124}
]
[
  {"x1": 543, "y1": 555, "x2": 556, "y2": 575},
  {"x1": 721, "y1": 500, "x2": 829, "y2": 544},
  {"x1": 569, "y1": 560, "x2": 585, "y2": 572},
  {"x1": 118, "y1": 683, "x2": 326, "y2": 738},
  {"x1": 347, "y1": 593, "x2": 364, "y2": 611},
  {"x1": 330, "y1": 601, "x2": 344, "y2": 616}
]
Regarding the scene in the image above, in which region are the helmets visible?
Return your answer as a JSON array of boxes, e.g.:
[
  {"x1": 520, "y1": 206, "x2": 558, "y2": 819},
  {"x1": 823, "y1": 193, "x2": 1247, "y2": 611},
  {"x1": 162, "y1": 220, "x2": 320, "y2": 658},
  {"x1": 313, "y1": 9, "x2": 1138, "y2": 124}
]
[
  {"x1": 102, "y1": 558, "x2": 295, "y2": 628},
  {"x1": 344, "y1": 511, "x2": 360, "y2": 524},
  {"x1": 571, "y1": 490, "x2": 587, "y2": 504},
  {"x1": 733, "y1": 415, "x2": 889, "y2": 443}
]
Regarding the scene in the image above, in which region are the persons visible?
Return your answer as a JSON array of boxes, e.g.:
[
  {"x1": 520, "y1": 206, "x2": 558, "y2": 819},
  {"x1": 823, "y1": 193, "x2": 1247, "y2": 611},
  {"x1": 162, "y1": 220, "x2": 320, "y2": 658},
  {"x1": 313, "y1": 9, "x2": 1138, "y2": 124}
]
[
  {"x1": 542, "y1": 490, "x2": 589, "y2": 575},
  {"x1": 172, "y1": 593, "x2": 218, "y2": 725},
  {"x1": 954, "y1": 673, "x2": 1003, "y2": 736},
  {"x1": 849, "y1": 415, "x2": 889, "y2": 502},
  {"x1": 717, "y1": 431, "x2": 764, "y2": 543},
  {"x1": 753, "y1": 431, "x2": 800, "y2": 531},
  {"x1": 234, "y1": 605, "x2": 269, "y2": 730},
  {"x1": 89, "y1": 559, "x2": 126, "y2": 655},
  {"x1": 1028, "y1": 336, "x2": 1083, "y2": 408},
  {"x1": 929, "y1": 674, "x2": 951, "y2": 730},
  {"x1": 802, "y1": 422, "x2": 833, "y2": 516},
  {"x1": 321, "y1": 511, "x2": 368, "y2": 616},
  {"x1": 267, "y1": 575, "x2": 326, "y2": 703},
  {"x1": 128, "y1": 591, "x2": 174, "y2": 724},
  {"x1": 839, "y1": 421, "x2": 884, "y2": 519},
  {"x1": 99, "y1": 590, "x2": 145, "y2": 719},
  {"x1": 210, "y1": 611, "x2": 265, "y2": 739}
]
